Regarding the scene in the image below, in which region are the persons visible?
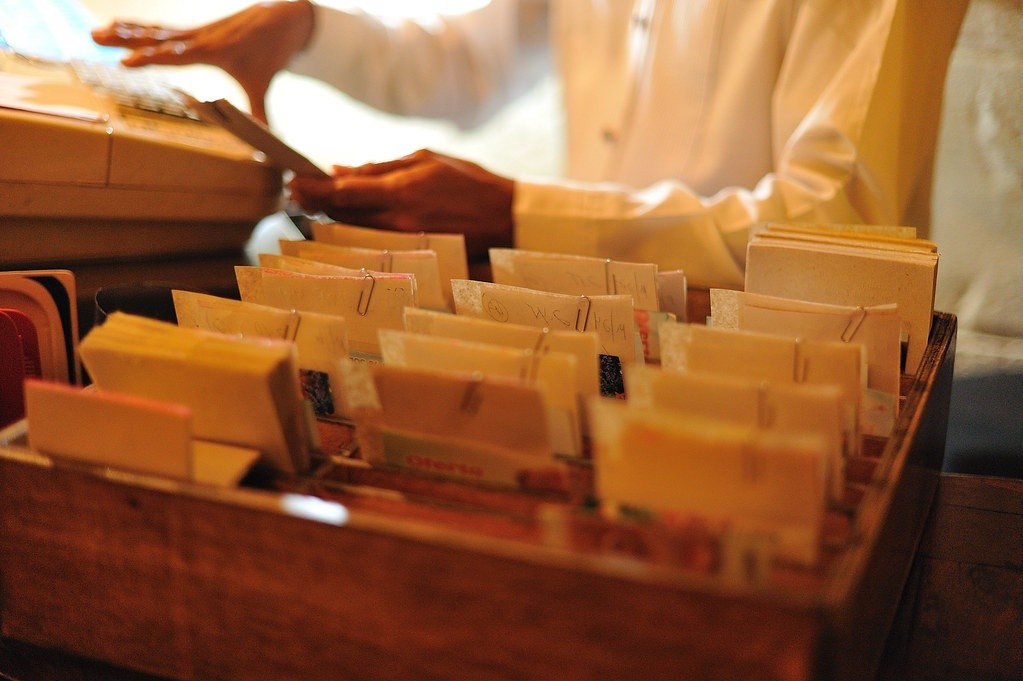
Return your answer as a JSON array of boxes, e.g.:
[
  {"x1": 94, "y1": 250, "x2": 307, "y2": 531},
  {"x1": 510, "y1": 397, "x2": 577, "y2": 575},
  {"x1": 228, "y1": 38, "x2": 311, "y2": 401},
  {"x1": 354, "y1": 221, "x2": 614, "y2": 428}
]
[{"x1": 91, "y1": 0, "x2": 971, "y2": 290}]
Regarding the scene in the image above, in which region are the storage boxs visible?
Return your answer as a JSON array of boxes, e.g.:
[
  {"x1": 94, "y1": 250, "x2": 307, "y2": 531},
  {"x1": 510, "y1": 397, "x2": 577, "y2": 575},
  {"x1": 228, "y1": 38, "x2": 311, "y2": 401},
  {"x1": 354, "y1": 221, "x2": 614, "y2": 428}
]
[{"x1": 1, "y1": 258, "x2": 958, "y2": 681}]
